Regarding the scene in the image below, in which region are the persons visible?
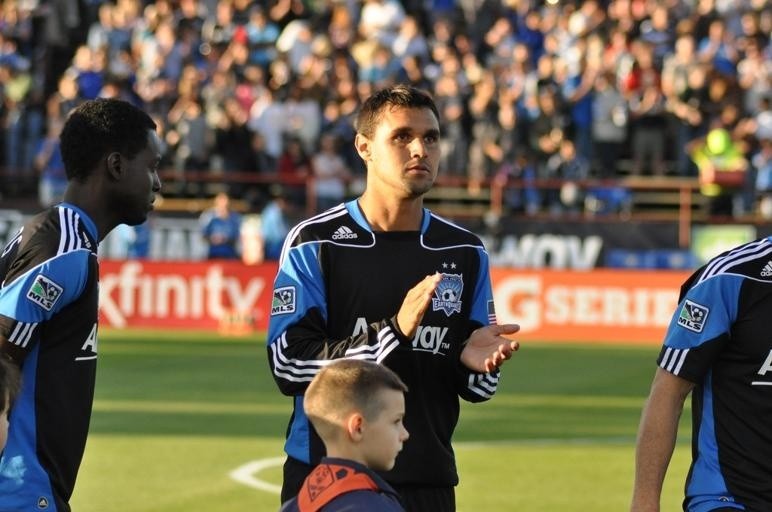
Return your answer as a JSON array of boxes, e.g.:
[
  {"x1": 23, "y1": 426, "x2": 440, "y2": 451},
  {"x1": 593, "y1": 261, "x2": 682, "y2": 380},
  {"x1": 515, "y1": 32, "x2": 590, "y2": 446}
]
[
  {"x1": 267, "y1": 84, "x2": 521, "y2": 512},
  {"x1": 634, "y1": 234, "x2": 771, "y2": 512},
  {"x1": 0, "y1": 97, "x2": 164, "y2": 510},
  {"x1": 276, "y1": 359, "x2": 415, "y2": 511},
  {"x1": 0, "y1": 0, "x2": 771, "y2": 261}
]
